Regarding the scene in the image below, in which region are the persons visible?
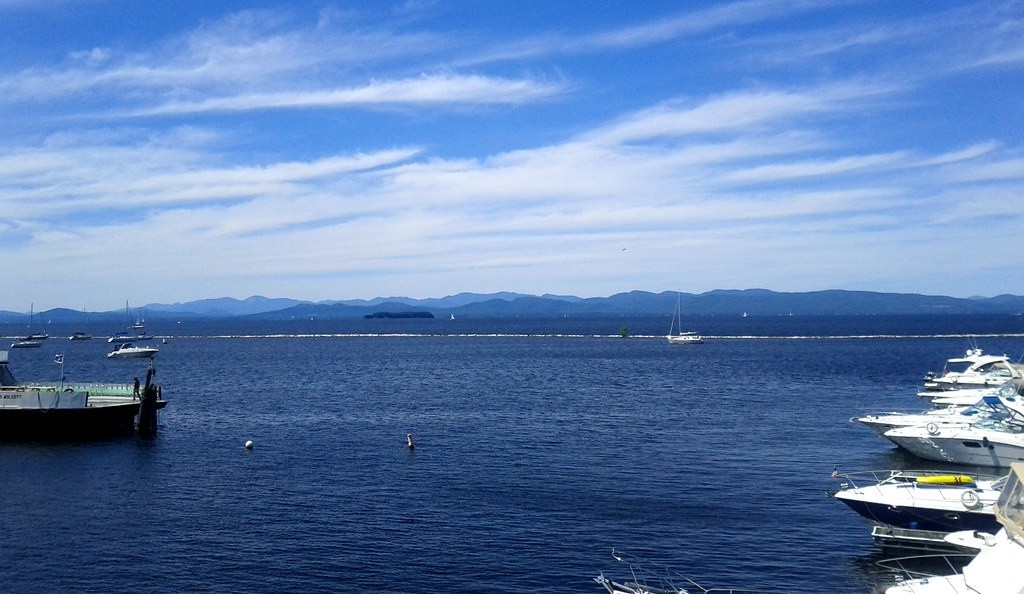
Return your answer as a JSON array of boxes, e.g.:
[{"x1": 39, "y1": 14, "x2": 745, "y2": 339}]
[{"x1": 132, "y1": 377, "x2": 141, "y2": 401}]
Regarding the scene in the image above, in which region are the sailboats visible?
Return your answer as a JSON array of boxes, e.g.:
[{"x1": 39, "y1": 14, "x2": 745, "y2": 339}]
[{"x1": 667, "y1": 289, "x2": 704, "y2": 344}]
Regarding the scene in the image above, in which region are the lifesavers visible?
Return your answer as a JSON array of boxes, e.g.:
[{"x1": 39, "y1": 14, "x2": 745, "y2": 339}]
[
  {"x1": 947, "y1": 404, "x2": 956, "y2": 415},
  {"x1": 960, "y1": 488, "x2": 980, "y2": 509},
  {"x1": 950, "y1": 375, "x2": 959, "y2": 381},
  {"x1": 926, "y1": 422, "x2": 939, "y2": 434}
]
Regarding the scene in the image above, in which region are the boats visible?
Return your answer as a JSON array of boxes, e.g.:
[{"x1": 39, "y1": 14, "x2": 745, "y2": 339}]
[
  {"x1": 163, "y1": 339, "x2": 167, "y2": 344},
  {"x1": 11, "y1": 336, "x2": 43, "y2": 348},
  {"x1": 107, "y1": 343, "x2": 160, "y2": 358},
  {"x1": 858, "y1": 348, "x2": 1024, "y2": 466},
  {"x1": 127, "y1": 316, "x2": 153, "y2": 340},
  {"x1": 33, "y1": 333, "x2": 48, "y2": 340},
  {"x1": 107, "y1": 332, "x2": 138, "y2": 342},
  {"x1": 872, "y1": 526, "x2": 989, "y2": 554},
  {"x1": 885, "y1": 463, "x2": 1024, "y2": 594},
  {"x1": 831, "y1": 469, "x2": 1009, "y2": 537},
  {"x1": 54, "y1": 353, "x2": 63, "y2": 364},
  {"x1": 0, "y1": 350, "x2": 168, "y2": 442},
  {"x1": 70, "y1": 332, "x2": 92, "y2": 340}
]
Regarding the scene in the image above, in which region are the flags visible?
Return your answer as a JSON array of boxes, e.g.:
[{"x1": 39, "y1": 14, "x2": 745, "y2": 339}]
[{"x1": 53, "y1": 354, "x2": 63, "y2": 363}]
